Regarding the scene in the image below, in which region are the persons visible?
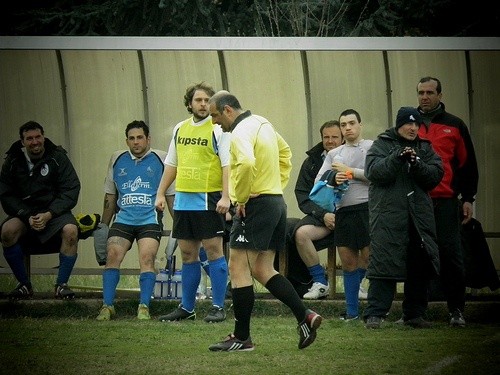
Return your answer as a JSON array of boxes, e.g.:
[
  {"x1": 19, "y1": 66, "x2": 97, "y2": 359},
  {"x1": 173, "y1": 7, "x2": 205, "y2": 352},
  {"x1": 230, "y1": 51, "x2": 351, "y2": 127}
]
[
  {"x1": 0, "y1": 121, "x2": 81, "y2": 300},
  {"x1": 154, "y1": 81, "x2": 236, "y2": 321},
  {"x1": 362, "y1": 106, "x2": 445, "y2": 329},
  {"x1": 287, "y1": 121, "x2": 368, "y2": 299},
  {"x1": 208, "y1": 90, "x2": 322, "y2": 351},
  {"x1": 395, "y1": 77, "x2": 479, "y2": 327},
  {"x1": 314, "y1": 109, "x2": 386, "y2": 322},
  {"x1": 94, "y1": 120, "x2": 176, "y2": 320}
]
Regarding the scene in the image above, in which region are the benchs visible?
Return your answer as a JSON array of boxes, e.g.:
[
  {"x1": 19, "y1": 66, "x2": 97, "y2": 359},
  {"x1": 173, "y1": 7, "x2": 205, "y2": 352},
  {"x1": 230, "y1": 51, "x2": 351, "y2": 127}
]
[{"x1": 0, "y1": 230, "x2": 177, "y2": 299}]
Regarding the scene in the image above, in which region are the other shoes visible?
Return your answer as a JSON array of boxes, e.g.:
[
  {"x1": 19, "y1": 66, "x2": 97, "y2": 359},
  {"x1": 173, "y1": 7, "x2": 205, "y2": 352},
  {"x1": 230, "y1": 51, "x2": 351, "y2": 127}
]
[
  {"x1": 340, "y1": 315, "x2": 359, "y2": 322},
  {"x1": 450, "y1": 312, "x2": 471, "y2": 327},
  {"x1": 403, "y1": 317, "x2": 433, "y2": 326},
  {"x1": 366, "y1": 317, "x2": 380, "y2": 328}
]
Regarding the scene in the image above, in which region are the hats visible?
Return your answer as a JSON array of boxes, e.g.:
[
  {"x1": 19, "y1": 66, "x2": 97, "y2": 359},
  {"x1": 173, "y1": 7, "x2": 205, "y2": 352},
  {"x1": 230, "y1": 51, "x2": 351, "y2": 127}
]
[{"x1": 395, "y1": 107, "x2": 422, "y2": 127}]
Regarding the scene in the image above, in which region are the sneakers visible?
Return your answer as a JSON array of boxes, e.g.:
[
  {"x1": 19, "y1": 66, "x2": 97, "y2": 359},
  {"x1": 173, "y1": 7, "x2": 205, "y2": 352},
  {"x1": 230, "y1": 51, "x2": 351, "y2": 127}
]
[
  {"x1": 138, "y1": 303, "x2": 150, "y2": 321},
  {"x1": 210, "y1": 333, "x2": 254, "y2": 352},
  {"x1": 296, "y1": 309, "x2": 323, "y2": 349},
  {"x1": 96, "y1": 305, "x2": 116, "y2": 321},
  {"x1": 10, "y1": 282, "x2": 33, "y2": 299},
  {"x1": 55, "y1": 283, "x2": 74, "y2": 300},
  {"x1": 204, "y1": 305, "x2": 226, "y2": 322},
  {"x1": 158, "y1": 303, "x2": 197, "y2": 322},
  {"x1": 303, "y1": 281, "x2": 330, "y2": 299}
]
